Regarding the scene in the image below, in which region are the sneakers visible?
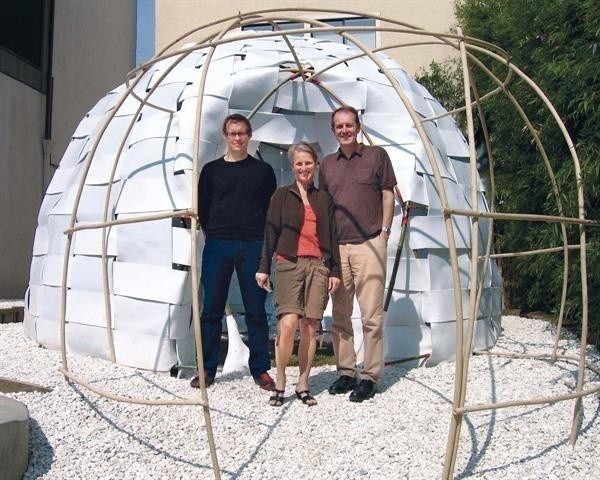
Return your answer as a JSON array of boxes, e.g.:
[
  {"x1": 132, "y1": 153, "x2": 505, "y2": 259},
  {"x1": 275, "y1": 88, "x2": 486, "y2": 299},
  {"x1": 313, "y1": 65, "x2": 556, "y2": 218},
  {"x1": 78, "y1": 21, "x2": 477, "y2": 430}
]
[
  {"x1": 190, "y1": 371, "x2": 216, "y2": 388},
  {"x1": 254, "y1": 372, "x2": 275, "y2": 391}
]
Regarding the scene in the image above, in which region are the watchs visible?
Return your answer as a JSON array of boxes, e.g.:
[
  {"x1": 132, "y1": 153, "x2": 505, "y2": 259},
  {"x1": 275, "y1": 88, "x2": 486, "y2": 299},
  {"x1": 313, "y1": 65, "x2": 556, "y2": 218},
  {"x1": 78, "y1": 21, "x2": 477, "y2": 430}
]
[{"x1": 380, "y1": 226, "x2": 391, "y2": 234}]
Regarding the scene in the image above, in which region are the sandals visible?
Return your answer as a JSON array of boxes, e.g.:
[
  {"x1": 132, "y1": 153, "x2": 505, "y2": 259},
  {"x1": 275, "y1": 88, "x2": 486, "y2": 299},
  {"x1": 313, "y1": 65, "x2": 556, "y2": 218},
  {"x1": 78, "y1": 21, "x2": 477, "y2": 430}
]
[
  {"x1": 270, "y1": 389, "x2": 284, "y2": 407},
  {"x1": 295, "y1": 390, "x2": 316, "y2": 406}
]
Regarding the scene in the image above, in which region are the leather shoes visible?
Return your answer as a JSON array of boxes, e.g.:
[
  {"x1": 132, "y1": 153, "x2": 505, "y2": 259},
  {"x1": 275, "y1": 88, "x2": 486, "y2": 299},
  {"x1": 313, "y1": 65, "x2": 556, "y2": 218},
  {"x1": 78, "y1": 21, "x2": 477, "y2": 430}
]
[
  {"x1": 329, "y1": 375, "x2": 358, "y2": 395},
  {"x1": 349, "y1": 380, "x2": 377, "y2": 402}
]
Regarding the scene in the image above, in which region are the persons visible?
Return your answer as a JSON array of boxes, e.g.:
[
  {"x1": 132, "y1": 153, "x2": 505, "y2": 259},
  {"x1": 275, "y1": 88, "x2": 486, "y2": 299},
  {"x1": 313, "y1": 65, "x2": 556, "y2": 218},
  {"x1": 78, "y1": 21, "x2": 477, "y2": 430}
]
[
  {"x1": 191, "y1": 113, "x2": 279, "y2": 391},
  {"x1": 317, "y1": 105, "x2": 398, "y2": 403},
  {"x1": 255, "y1": 140, "x2": 342, "y2": 406}
]
